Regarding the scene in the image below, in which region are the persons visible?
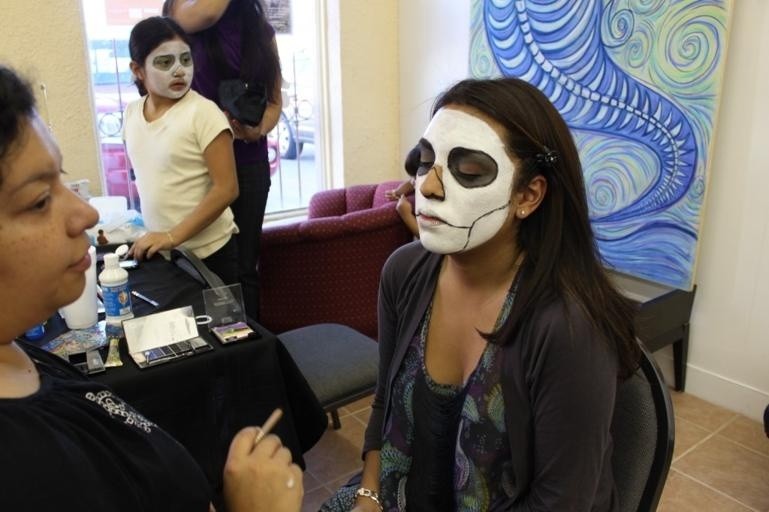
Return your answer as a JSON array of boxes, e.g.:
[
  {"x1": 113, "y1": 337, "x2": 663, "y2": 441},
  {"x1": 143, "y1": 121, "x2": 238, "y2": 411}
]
[
  {"x1": 1, "y1": 66, "x2": 304, "y2": 511},
  {"x1": 318, "y1": 76, "x2": 640, "y2": 511},
  {"x1": 384, "y1": 182, "x2": 420, "y2": 241},
  {"x1": 119, "y1": 16, "x2": 250, "y2": 314},
  {"x1": 159, "y1": 0, "x2": 282, "y2": 320}
]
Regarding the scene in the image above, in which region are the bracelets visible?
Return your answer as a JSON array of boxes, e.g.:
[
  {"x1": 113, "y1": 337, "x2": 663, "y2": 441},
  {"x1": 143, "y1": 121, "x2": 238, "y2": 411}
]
[
  {"x1": 351, "y1": 486, "x2": 386, "y2": 507},
  {"x1": 165, "y1": 231, "x2": 176, "y2": 246}
]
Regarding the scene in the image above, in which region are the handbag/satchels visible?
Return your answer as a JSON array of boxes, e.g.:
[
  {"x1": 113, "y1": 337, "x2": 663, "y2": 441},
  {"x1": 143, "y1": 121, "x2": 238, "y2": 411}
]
[{"x1": 207, "y1": 7, "x2": 278, "y2": 127}]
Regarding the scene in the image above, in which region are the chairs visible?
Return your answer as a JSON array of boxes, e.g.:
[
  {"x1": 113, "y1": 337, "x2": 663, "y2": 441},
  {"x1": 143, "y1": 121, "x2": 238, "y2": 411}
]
[{"x1": 263, "y1": 183, "x2": 411, "y2": 339}]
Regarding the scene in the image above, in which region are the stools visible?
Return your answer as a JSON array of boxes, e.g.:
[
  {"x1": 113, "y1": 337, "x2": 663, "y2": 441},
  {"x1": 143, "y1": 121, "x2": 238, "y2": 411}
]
[{"x1": 273, "y1": 325, "x2": 380, "y2": 430}]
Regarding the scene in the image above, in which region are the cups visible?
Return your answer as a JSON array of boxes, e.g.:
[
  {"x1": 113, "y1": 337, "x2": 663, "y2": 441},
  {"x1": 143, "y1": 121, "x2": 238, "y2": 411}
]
[{"x1": 56, "y1": 245, "x2": 97, "y2": 329}]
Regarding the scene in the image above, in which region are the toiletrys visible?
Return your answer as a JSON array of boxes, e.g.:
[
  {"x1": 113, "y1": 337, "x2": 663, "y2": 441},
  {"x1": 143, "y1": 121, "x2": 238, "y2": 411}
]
[
  {"x1": 104, "y1": 338, "x2": 124, "y2": 368},
  {"x1": 61, "y1": 245, "x2": 99, "y2": 330}
]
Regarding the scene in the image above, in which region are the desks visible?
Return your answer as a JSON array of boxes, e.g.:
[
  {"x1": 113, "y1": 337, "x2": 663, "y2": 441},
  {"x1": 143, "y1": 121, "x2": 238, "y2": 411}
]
[
  {"x1": 21, "y1": 222, "x2": 328, "y2": 474},
  {"x1": 607, "y1": 272, "x2": 697, "y2": 394}
]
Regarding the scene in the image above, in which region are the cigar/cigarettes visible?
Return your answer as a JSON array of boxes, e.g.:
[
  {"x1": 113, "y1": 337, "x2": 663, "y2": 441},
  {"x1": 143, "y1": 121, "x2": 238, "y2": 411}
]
[{"x1": 251, "y1": 408, "x2": 283, "y2": 446}]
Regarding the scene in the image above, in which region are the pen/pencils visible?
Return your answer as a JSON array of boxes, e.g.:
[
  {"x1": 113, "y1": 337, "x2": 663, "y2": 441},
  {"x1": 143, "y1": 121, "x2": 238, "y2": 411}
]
[{"x1": 131, "y1": 291, "x2": 159, "y2": 307}]
